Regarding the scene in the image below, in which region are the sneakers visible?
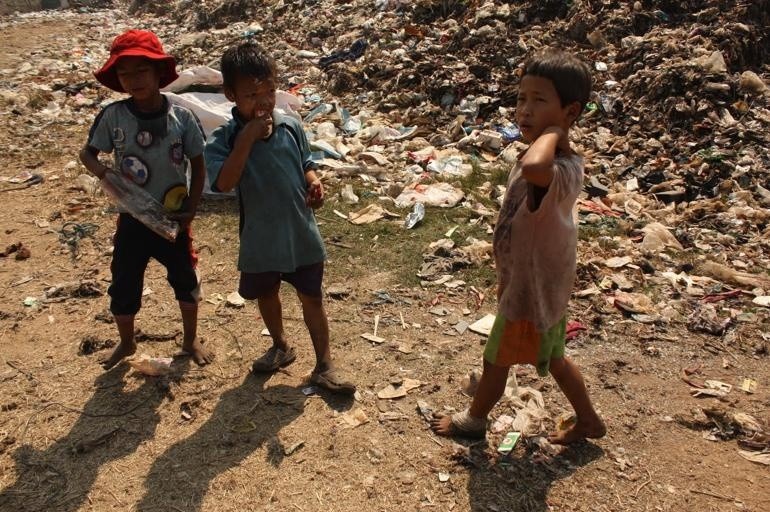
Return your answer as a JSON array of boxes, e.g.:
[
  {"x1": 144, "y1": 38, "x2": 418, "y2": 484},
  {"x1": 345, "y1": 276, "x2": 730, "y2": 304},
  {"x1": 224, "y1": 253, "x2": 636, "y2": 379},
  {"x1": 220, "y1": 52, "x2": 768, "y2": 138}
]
[
  {"x1": 310, "y1": 368, "x2": 356, "y2": 395},
  {"x1": 253, "y1": 346, "x2": 296, "y2": 371}
]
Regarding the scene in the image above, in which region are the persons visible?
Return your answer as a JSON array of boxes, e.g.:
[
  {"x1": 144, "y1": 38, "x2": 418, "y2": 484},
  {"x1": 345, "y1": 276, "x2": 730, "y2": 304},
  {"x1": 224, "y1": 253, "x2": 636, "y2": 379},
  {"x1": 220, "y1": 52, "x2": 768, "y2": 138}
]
[
  {"x1": 79, "y1": 29, "x2": 218, "y2": 372},
  {"x1": 429, "y1": 48, "x2": 608, "y2": 448},
  {"x1": 200, "y1": 41, "x2": 357, "y2": 398}
]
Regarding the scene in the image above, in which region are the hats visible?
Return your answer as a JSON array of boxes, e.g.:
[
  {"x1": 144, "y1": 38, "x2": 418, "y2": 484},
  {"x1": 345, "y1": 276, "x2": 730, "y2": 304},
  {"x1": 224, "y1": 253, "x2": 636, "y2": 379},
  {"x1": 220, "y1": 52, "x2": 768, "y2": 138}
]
[{"x1": 92, "y1": 29, "x2": 179, "y2": 93}]
